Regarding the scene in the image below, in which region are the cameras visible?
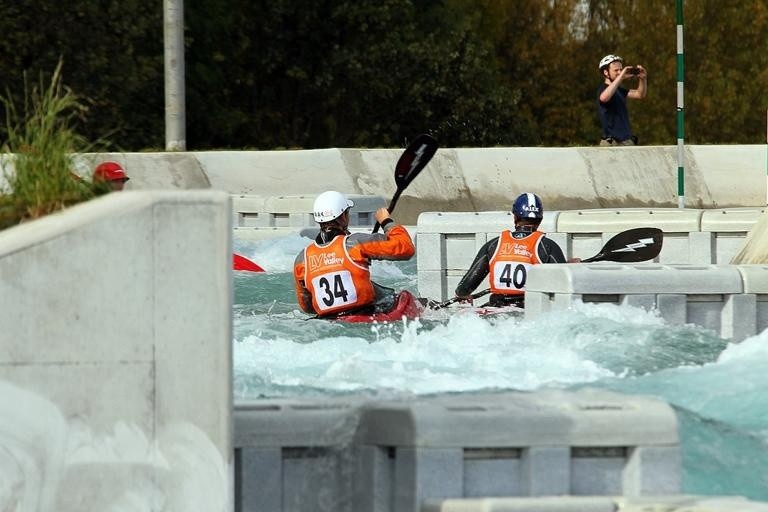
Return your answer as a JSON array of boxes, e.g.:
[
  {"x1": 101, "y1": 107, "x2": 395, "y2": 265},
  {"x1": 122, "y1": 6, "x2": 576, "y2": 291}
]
[{"x1": 630, "y1": 68, "x2": 640, "y2": 75}]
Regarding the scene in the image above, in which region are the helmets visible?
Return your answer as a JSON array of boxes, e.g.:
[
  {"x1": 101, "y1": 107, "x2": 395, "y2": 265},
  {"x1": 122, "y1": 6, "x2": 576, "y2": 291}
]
[
  {"x1": 599, "y1": 54, "x2": 623, "y2": 70},
  {"x1": 93, "y1": 162, "x2": 130, "y2": 183},
  {"x1": 313, "y1": 190, "x2": 355, "y2": 223},
  {"x1": 512, "y1": 194, "x2": 543, "y2": 222}
]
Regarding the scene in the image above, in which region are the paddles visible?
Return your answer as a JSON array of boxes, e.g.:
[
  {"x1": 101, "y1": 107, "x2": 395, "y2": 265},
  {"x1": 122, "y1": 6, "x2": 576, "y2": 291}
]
[
  {"x1": 69, "y1": 170, "x2": 264, "y2": 270},
  {"x1": 373, "y1": 135, "x2": 439, "y2": 235},
  {"x1": 432, "y1": 228, "x2": 662, "y2": 308}
]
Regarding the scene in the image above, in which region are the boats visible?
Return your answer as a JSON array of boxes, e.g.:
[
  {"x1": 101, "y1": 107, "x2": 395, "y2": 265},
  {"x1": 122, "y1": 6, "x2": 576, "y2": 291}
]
[{"x1": 328, "y1": 290, "x2": 493, "y2": 321}]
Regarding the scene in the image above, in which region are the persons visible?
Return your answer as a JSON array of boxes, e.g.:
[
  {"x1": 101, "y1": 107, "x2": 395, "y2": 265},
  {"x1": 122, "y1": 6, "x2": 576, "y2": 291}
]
[
  {"x1": 293, "y1": 189, "x2": 415, "y2": 328},
  {"x1": 597, "y1": 54, "x2": 648, "y2": 146},
  {"x1": 454, "y1": 192, "x2": 582, "y2": 310},
  {"x1": 92, "y1": 161, "x2": 130, "y2": 199}
]
